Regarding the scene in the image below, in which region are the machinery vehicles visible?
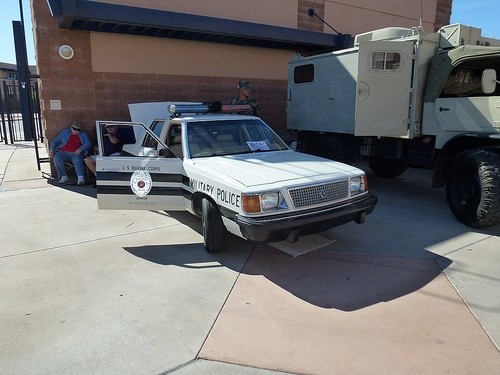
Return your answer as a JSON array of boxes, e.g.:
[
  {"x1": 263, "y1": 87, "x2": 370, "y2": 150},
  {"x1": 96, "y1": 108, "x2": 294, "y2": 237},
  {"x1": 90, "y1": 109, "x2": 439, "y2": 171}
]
[{"x1": 287, "y1": 23, "x2": 500, "y2": 227}]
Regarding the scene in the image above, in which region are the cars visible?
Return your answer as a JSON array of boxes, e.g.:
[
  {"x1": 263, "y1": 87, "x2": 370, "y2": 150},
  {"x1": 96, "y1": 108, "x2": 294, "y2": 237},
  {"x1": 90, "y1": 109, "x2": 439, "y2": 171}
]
[{"x1": 96, "y1": 102, "x2": 378, "y2": 252}]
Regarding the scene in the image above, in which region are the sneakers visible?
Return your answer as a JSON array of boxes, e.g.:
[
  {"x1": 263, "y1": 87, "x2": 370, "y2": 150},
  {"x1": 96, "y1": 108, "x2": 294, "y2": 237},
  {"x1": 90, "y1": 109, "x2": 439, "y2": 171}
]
[
  {"x1": 59, "y1": 176, "x2": 69, "y2": 183},
  {"x1": 77, "y1": 176, "x2": 85, "y2": 185}
]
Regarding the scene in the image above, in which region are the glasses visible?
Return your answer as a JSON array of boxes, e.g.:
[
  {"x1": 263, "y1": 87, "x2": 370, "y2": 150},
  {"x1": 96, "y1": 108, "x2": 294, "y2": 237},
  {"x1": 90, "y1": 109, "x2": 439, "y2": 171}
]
[{"x1": 72, "y1": 127, "x2": 81, "y2": 131}]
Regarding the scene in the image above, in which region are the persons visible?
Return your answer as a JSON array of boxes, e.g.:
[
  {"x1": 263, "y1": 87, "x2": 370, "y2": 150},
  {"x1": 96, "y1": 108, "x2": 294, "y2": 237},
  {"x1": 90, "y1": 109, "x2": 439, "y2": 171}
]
[
  {"x1": 229, "y1": 80, "x2": 264, "y2": 121},
  {"x1": 85, "y1": 124, "x2": 124, "y2": 188},
  {"x1": 50, "y1": 121, "x2": 92, "y2": 186}
]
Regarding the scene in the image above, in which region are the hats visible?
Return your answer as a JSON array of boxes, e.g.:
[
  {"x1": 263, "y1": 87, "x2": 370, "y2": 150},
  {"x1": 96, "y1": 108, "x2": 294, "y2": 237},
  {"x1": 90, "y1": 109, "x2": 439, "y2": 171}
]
[
  {"x1": 239, "y1": 80, "x2": 252, "y2": 89},
  {"x1": 71, "y1": 121, "x2": 83, "y2": 129}
]
